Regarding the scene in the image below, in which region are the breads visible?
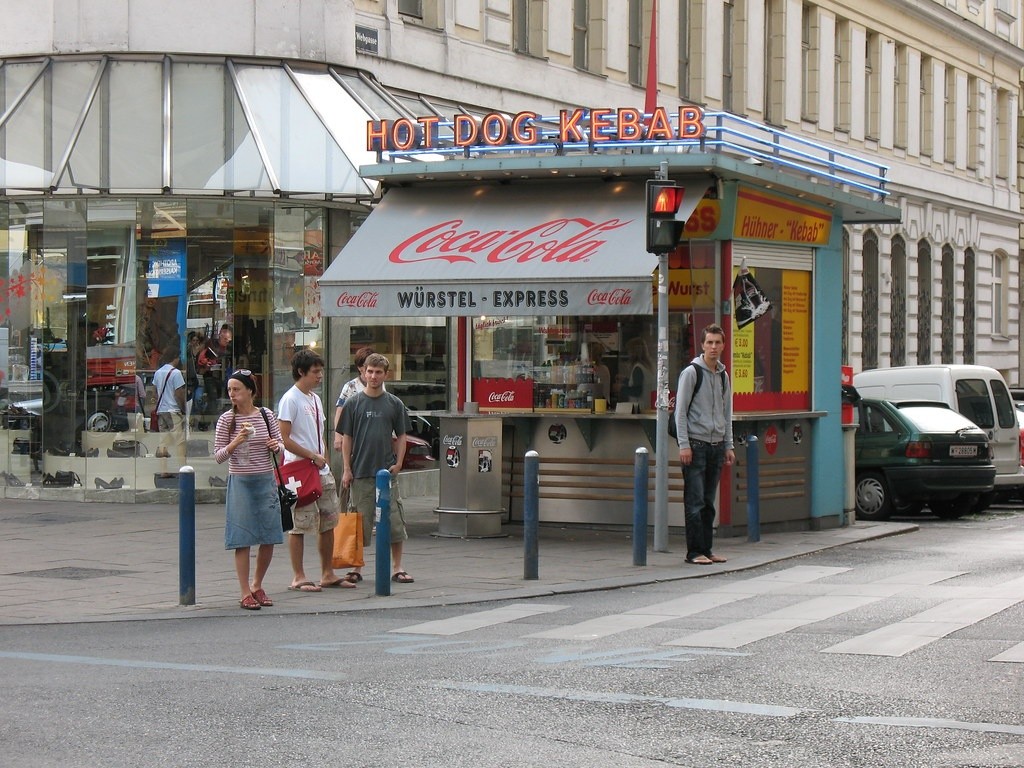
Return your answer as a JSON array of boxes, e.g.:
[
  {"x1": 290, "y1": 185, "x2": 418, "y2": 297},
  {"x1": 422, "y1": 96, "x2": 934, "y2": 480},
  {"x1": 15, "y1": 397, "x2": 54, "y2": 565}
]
[{"x1": 241, "y1": 423, "x2": 252, "y2": 429}]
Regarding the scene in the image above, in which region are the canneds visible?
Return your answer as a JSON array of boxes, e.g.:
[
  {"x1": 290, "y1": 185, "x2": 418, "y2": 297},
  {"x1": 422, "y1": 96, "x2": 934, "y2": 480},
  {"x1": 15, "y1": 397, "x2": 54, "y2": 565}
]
[{"x1": 546, "y1": 399, "x2": 552, "y2": 408}]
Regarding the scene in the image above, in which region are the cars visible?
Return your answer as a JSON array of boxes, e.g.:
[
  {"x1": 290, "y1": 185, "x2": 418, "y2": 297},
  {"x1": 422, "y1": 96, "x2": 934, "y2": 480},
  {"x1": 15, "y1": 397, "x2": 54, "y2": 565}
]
[
  {"x1": 1013, "y1": 400, "x2": 1024, "y2": 429},
  {"x1": 854, "y1": 397, "x2": 998, "y2": 522}
]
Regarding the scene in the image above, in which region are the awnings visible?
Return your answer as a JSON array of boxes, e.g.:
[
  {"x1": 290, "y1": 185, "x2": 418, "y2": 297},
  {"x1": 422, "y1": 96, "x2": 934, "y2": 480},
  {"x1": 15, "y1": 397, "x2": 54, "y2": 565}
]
[{"x1": 317, "y1": 174, "x2": 711, "y2": 317}]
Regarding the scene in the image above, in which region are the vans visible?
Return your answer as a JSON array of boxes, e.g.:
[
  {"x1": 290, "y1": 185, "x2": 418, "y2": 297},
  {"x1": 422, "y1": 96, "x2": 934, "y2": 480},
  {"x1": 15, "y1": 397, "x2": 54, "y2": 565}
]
[{"x1": 852, "y1": 364, "x2": 1024, "y2": 505}]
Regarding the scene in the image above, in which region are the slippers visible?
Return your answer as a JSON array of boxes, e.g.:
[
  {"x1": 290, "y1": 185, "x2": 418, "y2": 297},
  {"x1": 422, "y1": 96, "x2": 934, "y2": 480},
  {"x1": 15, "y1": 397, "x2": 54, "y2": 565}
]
[
  {"x1": 391, "y1": 572, "x2": 414, "y2": 583},
  {"x1": 344, "y1": 572, "x2": 363, "y2": 582},
  {"x1": 684, "y1": 555, "x2": 713, "y2": 565},
  {"x1": 288, "y1": 581, "x2": 321, "y2": 592},
  {"x1": 251, "y1": 589, "x2": 273, "y2": 606},
  {"x1": 707, "y1": 555, "x2": 727, "y2": 562},
  {"x1": 319, "y1": 578, "x2": 357, "y2": 588},
  {"x1": 239, "y1": 595, "x2": 262, "y2": 610}
]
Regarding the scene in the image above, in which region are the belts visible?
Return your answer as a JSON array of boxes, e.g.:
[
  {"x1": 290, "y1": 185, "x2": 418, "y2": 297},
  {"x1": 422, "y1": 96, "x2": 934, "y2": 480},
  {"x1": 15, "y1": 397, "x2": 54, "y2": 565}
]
[{"x1": 689, "y1": 437, "x2": 724, "y2": 446}]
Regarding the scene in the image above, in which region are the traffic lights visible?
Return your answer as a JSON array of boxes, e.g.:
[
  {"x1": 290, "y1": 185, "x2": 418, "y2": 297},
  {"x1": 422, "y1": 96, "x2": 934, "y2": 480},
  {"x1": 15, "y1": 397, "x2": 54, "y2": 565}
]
[{"x1": 644, "y1": 179, "x2": 685, "y2": 255}]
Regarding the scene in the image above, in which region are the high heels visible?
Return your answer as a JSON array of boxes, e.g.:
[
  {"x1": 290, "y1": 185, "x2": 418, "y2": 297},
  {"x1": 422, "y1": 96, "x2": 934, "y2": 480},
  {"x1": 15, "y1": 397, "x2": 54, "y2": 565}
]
[
  {"x1": 80, "y1": 447, "x2": 99, "y2": 457},
  {"x1": 1, "y1": 471, "x2": 27, "y2": 487},
  {"x1": 95, "y1": 476, "x2": 124, "y2": 489},
  {"x1": 209, "y1": 476, "x2": 226, "y2": 487}
]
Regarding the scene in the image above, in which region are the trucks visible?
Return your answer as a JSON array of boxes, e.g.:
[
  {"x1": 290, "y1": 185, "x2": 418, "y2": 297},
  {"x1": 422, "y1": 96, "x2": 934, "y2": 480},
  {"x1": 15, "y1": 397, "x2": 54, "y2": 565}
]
[{"x1": 87, "y1": 354, "x2": 136, "y2": 433}]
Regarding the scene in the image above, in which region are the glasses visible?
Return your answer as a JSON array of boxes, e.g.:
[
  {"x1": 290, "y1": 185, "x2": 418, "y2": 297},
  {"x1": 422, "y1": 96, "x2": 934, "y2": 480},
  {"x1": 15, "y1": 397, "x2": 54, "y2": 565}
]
[{"x1": 231, "y1": 369, "x2": 252, "y2": 376}]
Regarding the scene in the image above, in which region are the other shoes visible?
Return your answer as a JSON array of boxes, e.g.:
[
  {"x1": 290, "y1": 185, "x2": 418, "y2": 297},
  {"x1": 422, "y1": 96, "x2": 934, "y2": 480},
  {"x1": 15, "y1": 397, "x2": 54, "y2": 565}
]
[
  {"x1": 107, "y1": 448, "x2": 129, "y2": 457},
  {"x1": 40, "y1": 472, "x2": 56, "y2": 486},
  {"x1": 156, "y1": 447, "x2": 171, "y2": 458}
]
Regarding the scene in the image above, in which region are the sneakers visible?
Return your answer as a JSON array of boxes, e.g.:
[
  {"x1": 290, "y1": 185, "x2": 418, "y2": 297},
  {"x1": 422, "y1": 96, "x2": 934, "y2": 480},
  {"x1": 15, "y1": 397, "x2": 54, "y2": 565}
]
[{"x1": 102, "y1": 305, "x2": 117, "y2": 345}]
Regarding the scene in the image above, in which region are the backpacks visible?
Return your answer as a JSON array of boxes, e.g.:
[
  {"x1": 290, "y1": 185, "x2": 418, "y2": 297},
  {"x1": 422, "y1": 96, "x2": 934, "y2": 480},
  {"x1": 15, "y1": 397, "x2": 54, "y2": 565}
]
[{"x1": 667, "y1": 363, "x2": 725, "y2": 438}]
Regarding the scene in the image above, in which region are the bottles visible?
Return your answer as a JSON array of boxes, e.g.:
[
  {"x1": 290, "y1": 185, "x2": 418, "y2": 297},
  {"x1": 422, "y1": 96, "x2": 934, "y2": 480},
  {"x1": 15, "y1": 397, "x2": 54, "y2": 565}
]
[{"x1": 530, "y1": 360, "x2": 597, "y2": 411}]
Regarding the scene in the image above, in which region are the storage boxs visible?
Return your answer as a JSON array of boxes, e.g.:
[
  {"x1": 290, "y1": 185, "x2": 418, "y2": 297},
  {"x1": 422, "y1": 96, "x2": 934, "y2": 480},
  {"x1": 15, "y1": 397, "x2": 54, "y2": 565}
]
[{"x1": 471, "y1": 377, "x2": 533, "y2": 414}]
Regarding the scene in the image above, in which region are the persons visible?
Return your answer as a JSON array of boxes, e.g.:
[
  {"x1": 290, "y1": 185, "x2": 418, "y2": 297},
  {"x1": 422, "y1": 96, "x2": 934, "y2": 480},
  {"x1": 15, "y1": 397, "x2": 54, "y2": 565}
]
[
  {"x1": 214, "y1": 369, "x2": 285, "y2": 610},
  {"x1": 674, "y1": 325, "x2": 735, "y2": 564},
  {"x1": 119, "y1": 376, "x2": 146, "y2": 412},
  {"x1": 152, "y1": 347, "x2": 187, "y2": 479},
  {"x1": 187, "y1": 329, "x2": 233, "y2": 432},
  {"x1": 588, "y1": 342, "x2": 610, "y2": 403},
  {"x1": 613, "y1": 337, "x2": 656, "y2": 409},
  {"x1": 334, "y1": 347, "x2": 386, "y2": 452},
  {"x1": 277, "y1": 349, "x2": 357, "y2": 593},
  {"x1": 335, "y1": 353, "x2": 415, "y2": 583}
]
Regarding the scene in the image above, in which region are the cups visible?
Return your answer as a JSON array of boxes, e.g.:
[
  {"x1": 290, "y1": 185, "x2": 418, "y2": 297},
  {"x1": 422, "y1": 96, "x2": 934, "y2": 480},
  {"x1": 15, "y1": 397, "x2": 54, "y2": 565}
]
[{"x1": 594, "y1": 399, "x2": 606, "y2": 414}]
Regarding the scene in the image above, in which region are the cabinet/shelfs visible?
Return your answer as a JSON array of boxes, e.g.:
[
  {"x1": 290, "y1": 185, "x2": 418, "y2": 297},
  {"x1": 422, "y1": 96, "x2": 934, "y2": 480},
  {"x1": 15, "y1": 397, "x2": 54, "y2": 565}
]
[{"x1": 534, "y1": 382, "x2": 604, "y2": 414}]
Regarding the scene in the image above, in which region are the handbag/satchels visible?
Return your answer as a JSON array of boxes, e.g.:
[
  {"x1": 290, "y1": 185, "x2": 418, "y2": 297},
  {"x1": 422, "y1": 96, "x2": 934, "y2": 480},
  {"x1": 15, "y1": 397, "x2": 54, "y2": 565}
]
[
  {"x1": 112, "y1": 440, "x2": 148, "y2": 457},
  {"x1": 153, "y1": 473, "x2": 177, "y2": 488},
  {"x1": 48, "y1": 440, "x2": 76, "y2": 456},
  {"x1": 186, "y1": 438, "x2": 209, "y2": 456},
  {"x1": 55, "y1": 471, "x2": 83, "y2": 488},
  {"x1": 150, "y1": 409, "x2": 159, "y2": 432},
  {"x1": 279, "y1": 488, "x2": 298, "y2": 533},
  {"x1": 274, "y1": 458, "x2": 323, "y2": 508},
  {"x1": 330, "y1": 484, "x2": 365, "y2": 569}
]
